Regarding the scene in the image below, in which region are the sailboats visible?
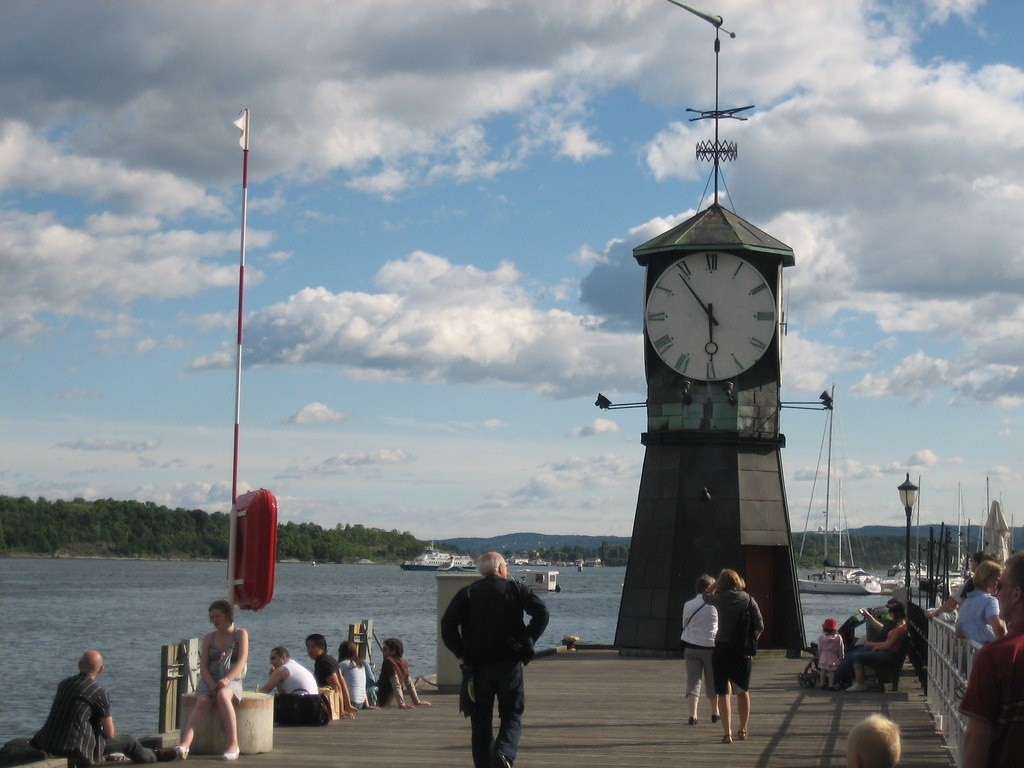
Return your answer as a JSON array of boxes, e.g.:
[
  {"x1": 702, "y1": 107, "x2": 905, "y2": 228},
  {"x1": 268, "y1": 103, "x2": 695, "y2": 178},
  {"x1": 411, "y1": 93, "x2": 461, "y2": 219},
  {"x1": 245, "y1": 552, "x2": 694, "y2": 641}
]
[
  {"x1": 880, "y1": 475, "x2": 1014, "y2": 599},
  {"x1": 796, "y1": 384, "x2": 882, "y2": 595}
]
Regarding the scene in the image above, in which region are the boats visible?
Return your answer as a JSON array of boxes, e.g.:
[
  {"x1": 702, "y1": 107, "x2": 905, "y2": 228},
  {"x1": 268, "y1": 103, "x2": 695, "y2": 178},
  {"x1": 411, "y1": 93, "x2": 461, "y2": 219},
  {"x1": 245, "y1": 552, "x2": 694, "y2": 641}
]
[
  {"x1": 514, "y1": 569, "x2": 559, "y2": 592},
  {"x1": 398, "y1": 539, "x2": 477, "y2": 572}
]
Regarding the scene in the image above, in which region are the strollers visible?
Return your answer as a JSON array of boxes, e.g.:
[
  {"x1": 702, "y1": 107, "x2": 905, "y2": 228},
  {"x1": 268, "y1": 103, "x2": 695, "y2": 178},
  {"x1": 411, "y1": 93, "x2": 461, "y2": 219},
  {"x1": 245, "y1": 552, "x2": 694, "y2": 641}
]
[{"x1": 797, "y1": 610, "x2": 867, "y2": 688}]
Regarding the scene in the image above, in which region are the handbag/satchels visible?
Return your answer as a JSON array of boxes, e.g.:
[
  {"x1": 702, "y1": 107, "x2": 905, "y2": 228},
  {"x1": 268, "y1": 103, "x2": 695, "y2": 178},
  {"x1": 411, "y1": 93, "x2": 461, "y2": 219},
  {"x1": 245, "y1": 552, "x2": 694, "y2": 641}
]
[
  {"x1": 738, "y1": 597, "x2": 756, "y2": 656},
  {"x1": 319, "y1": 686, "x2": 341, "y2": 720},
  {"x1": 273, "y1": 688, "x2": 331, "y2": 727},
  {"x1": 458, "y1": 663, "x2": 478, "y2": 718},
  {"x1": 218, "y1": 651, "x2": 248, "y2": 679}
]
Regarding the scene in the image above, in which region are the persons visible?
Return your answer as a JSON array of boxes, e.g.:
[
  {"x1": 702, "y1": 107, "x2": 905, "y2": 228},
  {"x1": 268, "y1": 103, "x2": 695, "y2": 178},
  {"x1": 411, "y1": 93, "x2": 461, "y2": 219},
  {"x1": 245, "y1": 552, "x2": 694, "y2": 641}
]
[
  {"x1": 306, "y1": 634, "x2": 359, "y2": 719},
  {"x1": 847, "y1": 712, "x2": 901, "y2": 768},
  {"x1": 259, "y1": 646, "x2": 319, "y2": 695},
  {"x1": 817, "y1": 619, "x2": 844, "y2": 691},
  {"x1": 845, "y1": 599, "x2": 907, "y2": 691},
  {"x1": 680, "y1": 569, "x2": 764, "y2": 743},
  {"x1": 361, "y1": 638, "x2": 431, "y2": 709},
  {"x1": 338, "y1": 640, "x2": 381, "y2": 711},
  {"x1": 925, "y1": 550, "x2": 1024, "y2": 768},
  {"x1": 174, "y1": 599, "x2": 248, "y2": 760},
  {"x1": 441, "y1": 552, "x2": 548, "y2": 768},
  {"x1": 30, "y1": 650, "x2": 179, "y2": 765}
]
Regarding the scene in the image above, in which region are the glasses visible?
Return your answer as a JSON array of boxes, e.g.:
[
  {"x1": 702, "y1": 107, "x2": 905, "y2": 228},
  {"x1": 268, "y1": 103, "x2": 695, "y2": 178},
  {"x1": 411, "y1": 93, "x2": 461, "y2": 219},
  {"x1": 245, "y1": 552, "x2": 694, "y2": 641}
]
[{"x1": 994, "y1": 579, "x2": 1019, "y2": 596}]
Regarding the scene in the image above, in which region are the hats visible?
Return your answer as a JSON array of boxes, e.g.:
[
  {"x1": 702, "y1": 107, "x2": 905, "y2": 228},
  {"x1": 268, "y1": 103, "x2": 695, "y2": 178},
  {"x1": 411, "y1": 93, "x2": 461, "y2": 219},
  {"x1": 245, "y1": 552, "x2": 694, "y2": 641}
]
[
  {"x1": 822, "y1": 618, "x2": 838, "y2": 630},
  {"x1": 886, "y1": 602, "x2": 902, "y2": 610}
]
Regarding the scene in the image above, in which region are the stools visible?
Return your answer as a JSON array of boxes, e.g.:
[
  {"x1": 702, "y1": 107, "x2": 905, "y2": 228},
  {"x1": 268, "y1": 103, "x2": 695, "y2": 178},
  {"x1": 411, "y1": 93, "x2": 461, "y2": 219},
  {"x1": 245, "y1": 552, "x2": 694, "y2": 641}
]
[{"x1": 180, "y1": 688, "x2": 275, "y2": 756}]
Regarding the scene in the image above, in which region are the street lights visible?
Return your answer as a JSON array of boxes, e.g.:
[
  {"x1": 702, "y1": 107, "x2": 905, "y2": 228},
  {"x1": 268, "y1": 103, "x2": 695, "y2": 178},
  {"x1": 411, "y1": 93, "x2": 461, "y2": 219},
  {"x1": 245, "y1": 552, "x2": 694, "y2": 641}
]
[{"x1": 897, "y1": 473, "x2": 919, "y2": 599}]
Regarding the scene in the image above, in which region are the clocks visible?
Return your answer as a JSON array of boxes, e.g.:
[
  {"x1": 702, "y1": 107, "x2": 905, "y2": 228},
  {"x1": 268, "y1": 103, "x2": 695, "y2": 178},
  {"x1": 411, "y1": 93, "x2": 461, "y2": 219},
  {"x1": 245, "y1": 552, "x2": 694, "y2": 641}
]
[{"x1": 644, "y1": 250, "x2": 780, "y2": 384}]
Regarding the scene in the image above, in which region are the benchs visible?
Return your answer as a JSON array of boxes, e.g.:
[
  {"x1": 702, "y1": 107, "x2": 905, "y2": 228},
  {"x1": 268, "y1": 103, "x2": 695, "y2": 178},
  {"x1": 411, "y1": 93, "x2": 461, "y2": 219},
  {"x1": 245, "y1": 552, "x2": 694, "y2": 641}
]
[{"x1": 872, "y1": 636, "x2": 910, "y2": 692}]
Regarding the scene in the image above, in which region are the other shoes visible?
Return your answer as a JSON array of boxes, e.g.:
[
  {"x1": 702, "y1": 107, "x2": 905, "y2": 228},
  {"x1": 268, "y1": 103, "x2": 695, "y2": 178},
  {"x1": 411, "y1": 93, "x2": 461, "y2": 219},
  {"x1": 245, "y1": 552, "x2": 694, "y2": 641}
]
[
  {"x1": 738, "y1": 728, "x2": 748, "y2": 740},
  {"x1": 155, "y1": 749, "x2": 178, "y2": 761},
  {"x1": 712, "y1": 715, "x2": 720, "y2": 723},
  {"x1": 722, "y1": 733, "x2": 733, "y2": 743},
  {"x1": 174, "y1": 746, "x2": 190, "y2": 760},
  {"x1": 496, "y1": 753, "x2": 514, "y2": 768},
  {"x1": 846, "y1": 681, "x2": 864, "y2": 691},
  {"x1": 223, "y1": 747, "x2": 241, "y2": 761},
  {"x1": 689, "y1": 717, "x2": 698, "y2": 724}
]
